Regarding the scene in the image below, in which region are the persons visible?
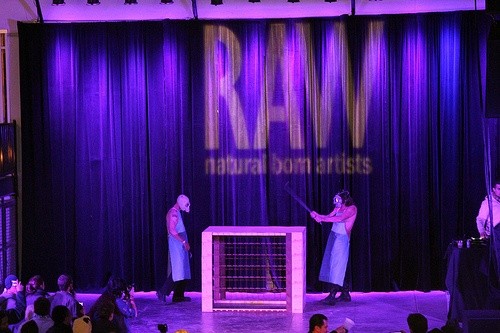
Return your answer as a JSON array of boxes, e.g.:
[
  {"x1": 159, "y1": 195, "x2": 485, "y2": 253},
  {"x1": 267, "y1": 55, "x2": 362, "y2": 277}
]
[
  {"x1": 50, "y1": 274, "x2": 84, "y2": 319},
  {"x1": 23, "y1": 275, "x2": 50, "y2": 304},
  {"x1": 0, "y1": 272, "x2": 500, "y2": 333},
  {"x1": 88, "y1": 276, "x2": 137, "y2": 333},
  {"x1": 476, "y1": 181, "x2": 500, "y2": 240},
  {"x1": 309, "y1": 190, "x2": 357, "y2": 305},
  {"x1": 156, "y1": 195, "x2": 193, "y2": 305}
]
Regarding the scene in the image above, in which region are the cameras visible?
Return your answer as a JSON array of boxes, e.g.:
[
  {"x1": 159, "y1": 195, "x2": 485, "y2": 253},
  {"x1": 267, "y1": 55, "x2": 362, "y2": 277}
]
[{"x1": 124, "y1": 284, "x2": 132, "y2": 299}]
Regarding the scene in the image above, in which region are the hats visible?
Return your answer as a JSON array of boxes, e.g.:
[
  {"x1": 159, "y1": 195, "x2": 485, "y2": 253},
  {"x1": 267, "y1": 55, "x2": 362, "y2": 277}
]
[{"x1": 4, "y1": 276, "x2": 17, "y2": 290}]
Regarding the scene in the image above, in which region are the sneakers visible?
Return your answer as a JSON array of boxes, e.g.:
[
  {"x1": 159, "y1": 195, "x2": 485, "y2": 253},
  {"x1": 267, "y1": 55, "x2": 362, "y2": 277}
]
[
  {"x1": 172, "y1": 297, "x2": 191, "y2": 302},
  {"x1": 156, "y1": 290, "x2": 167, "y2": 302}
]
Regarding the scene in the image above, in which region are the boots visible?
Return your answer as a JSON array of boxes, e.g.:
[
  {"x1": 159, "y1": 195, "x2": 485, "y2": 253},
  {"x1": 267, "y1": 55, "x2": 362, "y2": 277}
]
[
  {"x1": 318, "y1": 284, "x2": 338, "y2": 304},
  {"x1": 336, "y1": 287, "x2": 351, "y2": 302}
]
